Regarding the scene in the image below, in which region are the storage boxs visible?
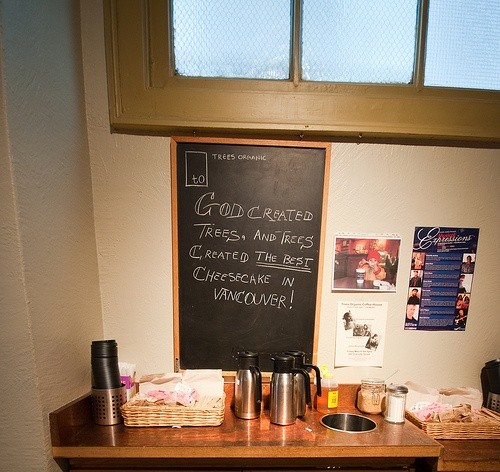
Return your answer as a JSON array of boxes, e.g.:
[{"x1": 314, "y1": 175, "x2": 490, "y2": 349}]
[
  {"x1": 121, "y1": 391, "x2": 226, "y2": 426},
  {"x1": 404, "y1": 406, "x2": 500, "y2": 439}
]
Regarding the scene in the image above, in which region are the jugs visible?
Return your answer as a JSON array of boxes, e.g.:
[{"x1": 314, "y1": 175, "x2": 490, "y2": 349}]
[
  {"x1": 282, "y1": 350, "x2": 321, "y2": 417},
  {"x1": 269, "y1": 354, "x2": 311, "y2": 426},
  {"x1": 235, "y1": 351, "x2": 262, "y2": 420}
]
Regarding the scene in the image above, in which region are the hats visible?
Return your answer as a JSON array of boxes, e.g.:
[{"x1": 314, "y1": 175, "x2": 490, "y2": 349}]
[{"x1": 367, "y1": 250, "x2": 380, "y2": 263}]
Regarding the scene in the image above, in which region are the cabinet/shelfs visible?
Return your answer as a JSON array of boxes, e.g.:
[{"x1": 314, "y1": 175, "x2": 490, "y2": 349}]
[
  {"x1": 437, "y1": 438, "x2": 500, "y2": 472},
  {"x1": 50, "y1": 381, "x2": 444, "y2": 472}
]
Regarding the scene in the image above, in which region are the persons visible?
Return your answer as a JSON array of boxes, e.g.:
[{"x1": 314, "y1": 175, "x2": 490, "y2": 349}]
[
  {"x1": 405, "y1": 305, "x2": 417, "y2": 324},
  {"x1": 407, "y1": 289, "x2": 420, "y2": 304},
  {"x1": 342, "y1": 309, "x2": 353, "y2": 330},
  {"x1": 366, "y1": 333, "x2": 379, "y2": 351},
  {"x1": 361, "y1": 324, "x2": 370, "y2": 336},
  {"x1": 354, "y1": 251, "x2": 386, "y2": 281},
  {"x1": 410, "y1": 270, "x2": 421, "y2": 286},
  {"x1": 411, "y1": 253, "x2": 423, "y2": 269},
  {"x1": 455, "y1": 310, "x2": 466, "y2": 328},
  {"x1": 456, "y1": 295, "x2": 470, "y2": 308},
  {"x1": 459, "y1": 275, "x2": 466, "y2": 293},
  {"x1": 461, "y1": 256, "x2": 474, "y2": 273}
]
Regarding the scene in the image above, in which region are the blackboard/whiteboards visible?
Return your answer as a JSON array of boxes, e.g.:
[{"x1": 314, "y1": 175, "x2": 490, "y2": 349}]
[{"x1": 170, "y1": 136, "x2": 332, "y2": 377}]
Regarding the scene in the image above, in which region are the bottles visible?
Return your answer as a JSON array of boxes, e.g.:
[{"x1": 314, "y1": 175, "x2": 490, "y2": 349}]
[
  {"x1": 384, "y1": 385, "x2": 408, "y2": 424},
  {"x1": 316, "y1": 379, "x2": 339, "y2": 413},
  {"x1": 357, "y1": 378, "x2": 387, "y2": 414}
]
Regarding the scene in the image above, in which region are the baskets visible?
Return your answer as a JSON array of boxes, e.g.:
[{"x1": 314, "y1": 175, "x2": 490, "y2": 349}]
[
  {"x1": 405, "y1": 406, "x2": 500, "y2": 439},
  {"x1": 119, "y1": 393, "x2": 226, "y2": 426}
]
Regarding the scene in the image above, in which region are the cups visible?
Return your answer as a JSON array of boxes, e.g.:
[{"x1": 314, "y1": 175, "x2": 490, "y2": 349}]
[{"x1": 356, "y1": 269, "x2": 365, "y2": 283}]
